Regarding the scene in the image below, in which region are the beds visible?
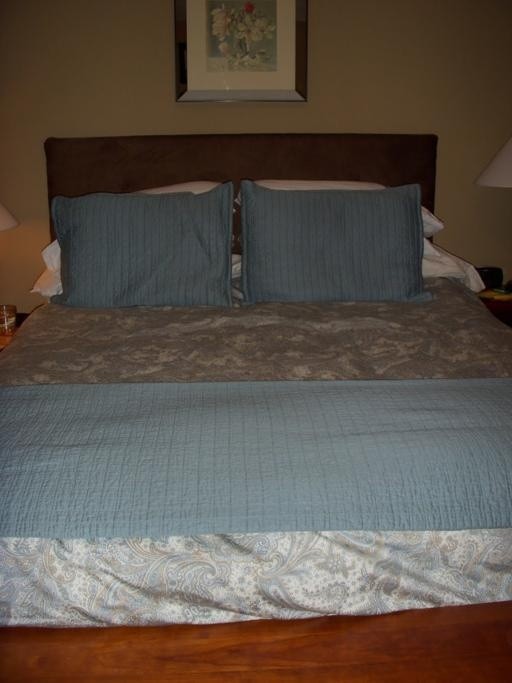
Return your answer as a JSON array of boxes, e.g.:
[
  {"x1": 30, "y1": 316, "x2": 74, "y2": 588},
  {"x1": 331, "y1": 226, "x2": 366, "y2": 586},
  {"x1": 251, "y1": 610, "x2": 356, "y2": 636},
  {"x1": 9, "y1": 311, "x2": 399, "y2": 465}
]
[{"x1": 0, "y1": 136, "x2": 512, "y2": 617}]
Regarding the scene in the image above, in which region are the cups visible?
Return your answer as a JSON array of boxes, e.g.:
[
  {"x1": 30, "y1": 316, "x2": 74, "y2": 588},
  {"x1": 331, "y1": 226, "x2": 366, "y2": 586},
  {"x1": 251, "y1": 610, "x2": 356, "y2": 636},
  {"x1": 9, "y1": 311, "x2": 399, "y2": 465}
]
[{"x1": 0, "y1": 304, "x2": 16, "y2": 336}]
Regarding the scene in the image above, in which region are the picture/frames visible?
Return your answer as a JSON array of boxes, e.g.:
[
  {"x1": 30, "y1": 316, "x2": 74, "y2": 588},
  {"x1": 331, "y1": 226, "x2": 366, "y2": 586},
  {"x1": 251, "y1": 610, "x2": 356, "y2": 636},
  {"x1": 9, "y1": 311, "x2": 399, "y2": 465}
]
[{"x1": 174, "y1": 0, "x2": 308, "y2": 102}]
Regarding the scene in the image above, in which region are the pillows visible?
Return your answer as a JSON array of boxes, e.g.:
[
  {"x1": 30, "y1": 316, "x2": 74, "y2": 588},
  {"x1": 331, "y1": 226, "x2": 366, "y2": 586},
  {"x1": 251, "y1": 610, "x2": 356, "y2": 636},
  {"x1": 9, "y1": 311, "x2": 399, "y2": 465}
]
[{"x1": 31, "y1": 179, "x2": 485, "y2": 309}]
